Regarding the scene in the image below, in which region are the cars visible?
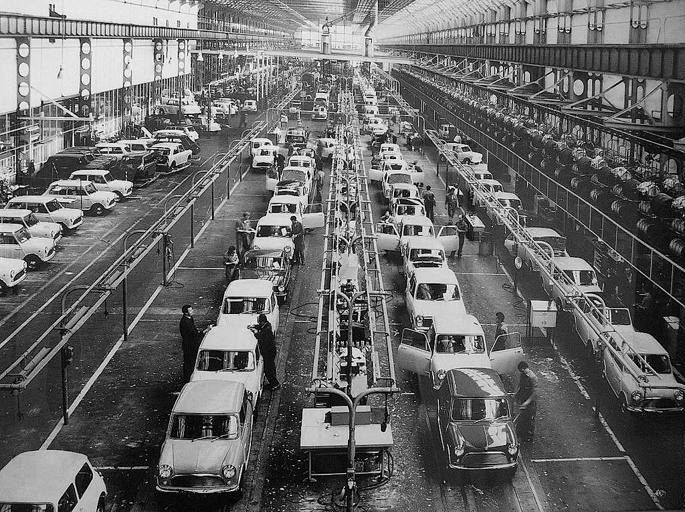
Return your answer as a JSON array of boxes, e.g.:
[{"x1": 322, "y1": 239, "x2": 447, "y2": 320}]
[
  {"x1": 318, "y1": 136, "x2": 355, "y2": 162},
  {"x1": 311, "y1": 78, "x2": 388, "y2": 135},
  {"x1": 602, "y1": 329, "x2": 685, "y2": 416},
  {"x1": 0, "y1": 450, "x2": 108, "y2": 512},
  {"x1": 574, "y1": 292, "x2": 637, "y2": 355},
  {"x1": 504, "y1": 225, "x2": 570, "y2": 271},
  {"x1": 540, "y1": 255, "x2": 604, "y2": 311}
]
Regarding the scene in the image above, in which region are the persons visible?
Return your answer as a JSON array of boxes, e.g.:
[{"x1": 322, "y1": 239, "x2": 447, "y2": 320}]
[
  {"x1": 179, "y1": 304, "x2": 212, "y2": 380},
  {"x1": 223, "y1": 245, "x2": 239, "y2": 282},
  {"x1": 234, "y1": 211, "x2": 254, "y2": 256},
  {"x1": 494, "y1": 311, "x2": 509, "y2": 350},
  {"x1": 515, "y1": 360, "x2": 539, "y2": 444},
  {"x1": 380, "y1": 210, "x2": 395, "y2": 258},
  {"x1": 238, "y1": 109, "x2": 248, "y2": 128},
  {"x1": 272, "y1": 140, "x2": 326, "y2": 202},
  {"x1": 601, "y1": 266, "x2": 653, "y2": 331},
  {"x1": 246, "y1": 314, "x2": 281, "y2": 392},
  {"x1": 421, "y1": 185, "x2": 469, "y2": 260},
  {"x1": 285, "y1": 216, "x2": 306, "y2": 266}
]
